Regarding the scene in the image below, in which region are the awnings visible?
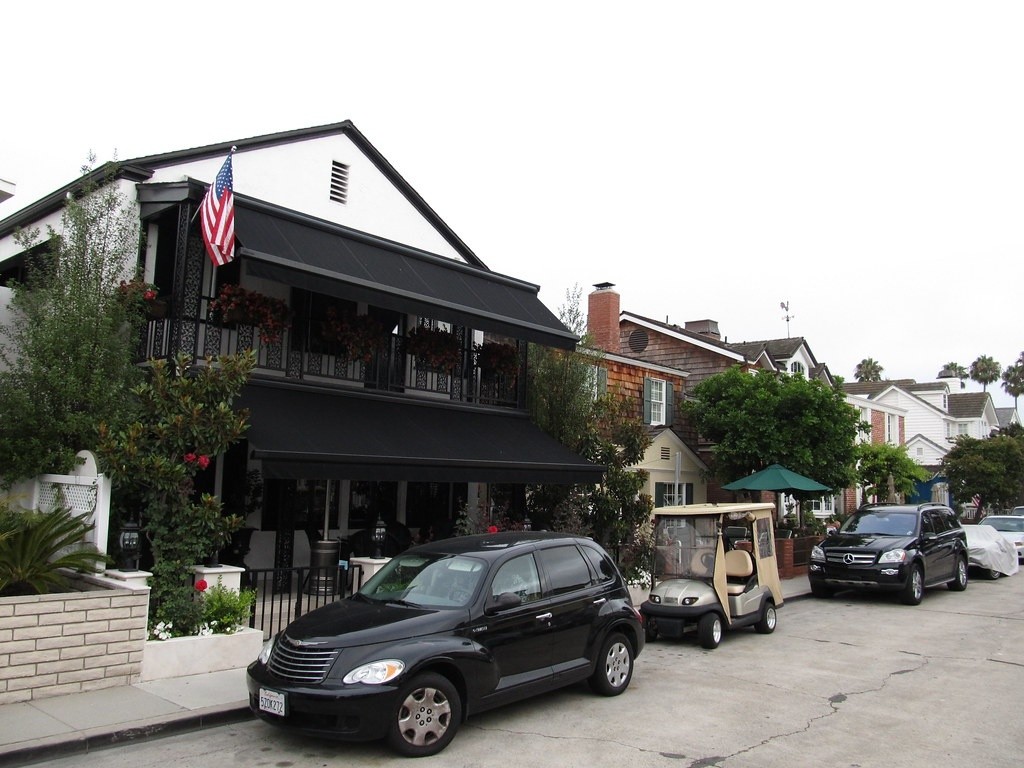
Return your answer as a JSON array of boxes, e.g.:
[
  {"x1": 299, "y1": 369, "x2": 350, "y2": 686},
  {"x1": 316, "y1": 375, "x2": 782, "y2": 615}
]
[
  {"x1": 233, "y1": 204, "x2": 581, "y2": 353},
  {"x1": 223, "y1": 384, "x2": 609, "y2": 483}
]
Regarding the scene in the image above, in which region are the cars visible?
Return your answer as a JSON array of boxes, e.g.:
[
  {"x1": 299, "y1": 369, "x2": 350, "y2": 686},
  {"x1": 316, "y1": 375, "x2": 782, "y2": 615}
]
[
  {"x1": 962, "y1": 524, "x2": 1001, "y2": 579},
  {"x1": 978, "y1": 515, "x2": 1024, "y2": 565},
  {"x1": 1012, "y1": 506, "x2": 1024, "y2": 516},
  {"x1": 242, "y1": 530, "x2": 647, "y2": 760}
]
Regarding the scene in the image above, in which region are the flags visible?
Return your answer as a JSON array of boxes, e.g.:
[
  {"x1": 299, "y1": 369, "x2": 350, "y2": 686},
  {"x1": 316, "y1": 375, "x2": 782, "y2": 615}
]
[{"x1": 199, "y1": 152, "x2": 235, "y2": 266}]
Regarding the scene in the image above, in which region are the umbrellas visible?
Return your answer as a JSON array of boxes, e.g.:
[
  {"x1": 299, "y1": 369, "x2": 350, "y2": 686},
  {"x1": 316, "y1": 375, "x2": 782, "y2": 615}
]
[{"x1": 720, "y1": 464, "x2": 834, "y2": 529}]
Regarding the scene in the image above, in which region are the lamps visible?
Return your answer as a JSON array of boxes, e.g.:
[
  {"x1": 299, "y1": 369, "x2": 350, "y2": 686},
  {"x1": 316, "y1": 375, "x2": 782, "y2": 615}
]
[
  {"x1": 522, "y1": 514, "x2": 532, "y2": 531},
  {"x1": 118, "y1": 510, "x2": 140, "y2": 572},
  {"x1": 368, "y1": 511, "x2": 386, "y2": 558}
]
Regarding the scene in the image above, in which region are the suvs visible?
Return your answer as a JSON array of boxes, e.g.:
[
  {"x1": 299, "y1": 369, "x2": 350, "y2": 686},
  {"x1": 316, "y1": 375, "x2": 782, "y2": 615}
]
[{"x1": 808, "y1": 501, "x2": 968, "y2": 606}]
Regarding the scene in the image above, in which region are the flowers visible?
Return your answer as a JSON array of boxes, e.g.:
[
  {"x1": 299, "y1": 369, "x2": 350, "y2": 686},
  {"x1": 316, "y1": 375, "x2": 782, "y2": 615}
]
[
  {"x1": 411, "y1": 325, "x2": 462, "y2": 371},
  {"x1": 209, "y1": 282, "x2": 295, "y2": 346},
  {"x1": 114, "y1": 276, "x2": 157, "y2": 299},
  {"x1": 146, "y1": 580, "x2": 245, "y2": 641},
  {"x1": 486, "y1": 524, "x2": 499, "y2": 534},
  {"x1": 474, "y1": 339, "x2": 521, "y2": 389},
  {"x1": 183, "y1": 453, "x2": 209, "y2": 470},
  {"x1": 321, "y1": 303, "x2": 381, "y2": 361}
]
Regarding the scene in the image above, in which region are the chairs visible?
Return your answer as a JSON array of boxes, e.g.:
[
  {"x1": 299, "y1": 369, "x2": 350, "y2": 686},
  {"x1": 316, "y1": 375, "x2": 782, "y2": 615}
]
[
  {"x1": 689, "y1": 547, "x2": 715, "y2": 576},
  {"x1": 725, "y1": 550, "x2": 756, "y2": 596}
]
